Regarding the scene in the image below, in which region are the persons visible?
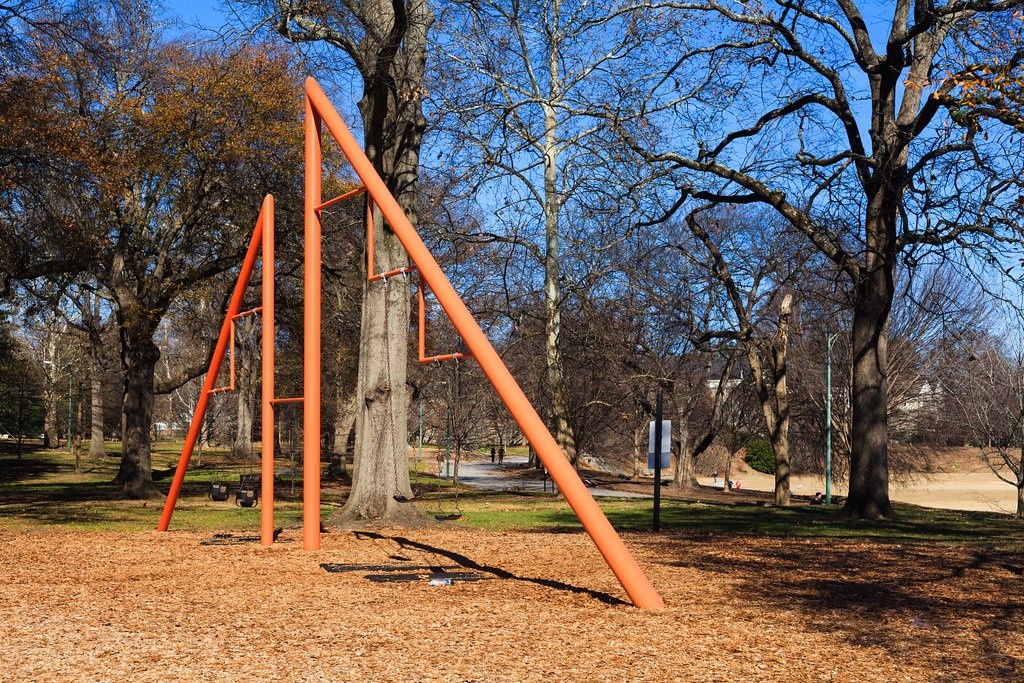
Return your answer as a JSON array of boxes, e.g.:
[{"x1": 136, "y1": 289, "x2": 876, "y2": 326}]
[
  {"x1": 490, "y1": 447, "x2": 496, "y2": 463},
  {"x1": 437, "y1": 451, "x2": 444, "y2": 473},
  {"x1": 711, "y1": 468, "x2": 718, "y2": 483},
  {"x1": 498, "y1": 446, "x2": 505, "y2": 465},
  {"x1": 810, "y1": 492, "x2": 822, "y2": 505},
  {"x1": 736, "y1": 480, "x2": 741, "y2": 489}
]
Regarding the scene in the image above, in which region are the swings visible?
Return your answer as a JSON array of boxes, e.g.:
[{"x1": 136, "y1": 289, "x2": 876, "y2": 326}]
[
  {"x1": 380, "y1": 268, "x2": 422, "y2": 504},
  {"x1": 234, "y1": 308, "x2": 260, "y2": 509},
  {"x1": 434, "y1": 351, "x2": 463, "y2": 521},
  {"x1": 207, "y1": 387, "x2": 231, "y2": 502}
]
